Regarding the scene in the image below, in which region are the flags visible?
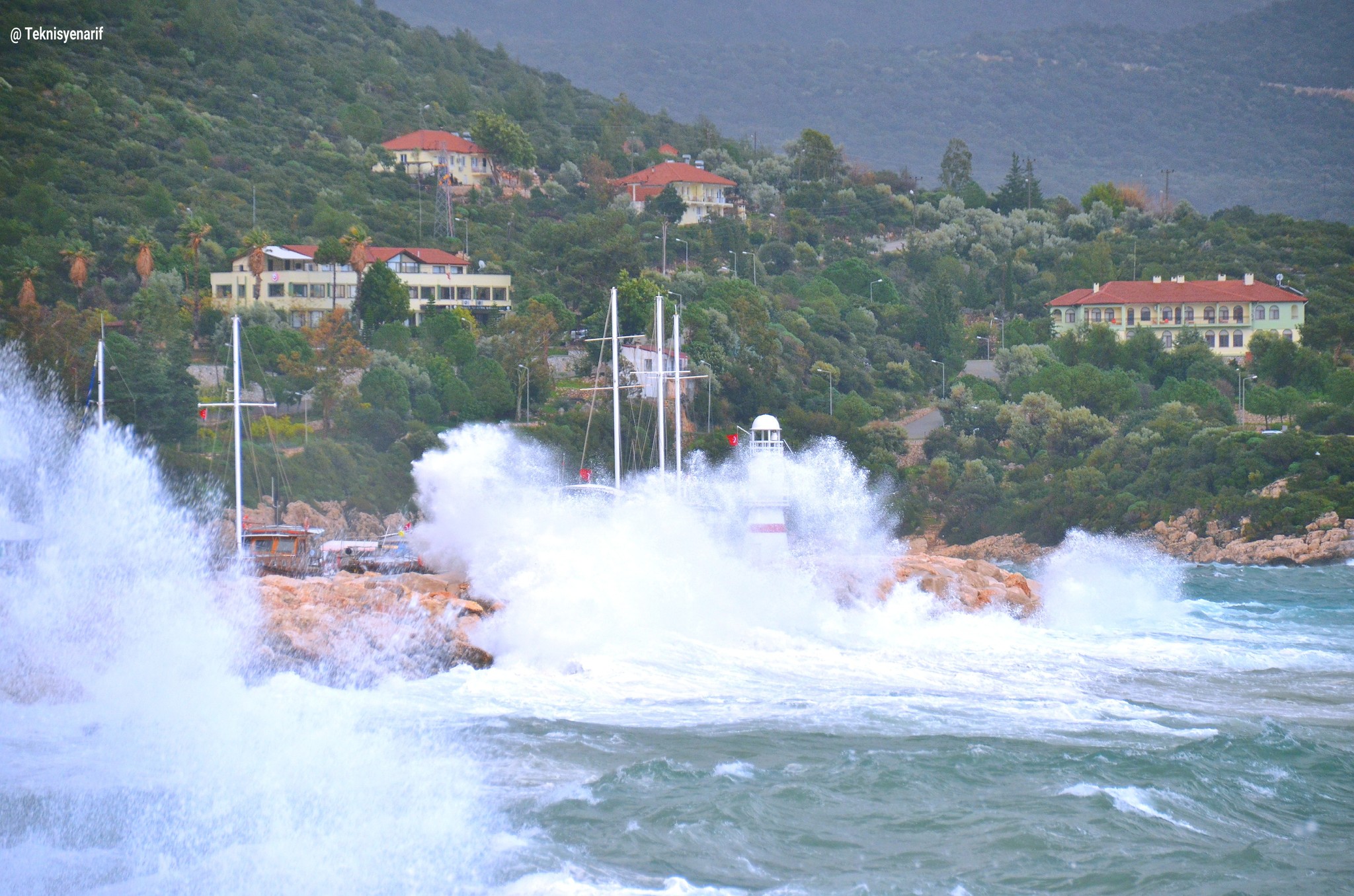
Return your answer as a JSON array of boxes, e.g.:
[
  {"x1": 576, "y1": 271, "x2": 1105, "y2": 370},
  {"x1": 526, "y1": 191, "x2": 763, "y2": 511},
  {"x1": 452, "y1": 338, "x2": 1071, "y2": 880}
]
[
  {"x1": 399, "y1": 530, "x2": 404, "y2": 536},
  {"x1": 402, "y1": 521, "x2": 411, "y2": 532},
  {"x1": 725, "y1": 433, "x2": 739, "y2": 446},
  {"x1": 580, "y1": 468, "x2": 592, "y2": 482},
  {"x1": 199, "y1": 408, "x2": 208, "y2": 419}
]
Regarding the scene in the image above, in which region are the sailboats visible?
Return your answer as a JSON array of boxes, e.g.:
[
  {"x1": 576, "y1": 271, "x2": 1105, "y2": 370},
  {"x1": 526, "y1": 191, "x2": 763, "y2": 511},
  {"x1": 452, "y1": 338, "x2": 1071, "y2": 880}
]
[
  {"x1": 500, "y1": 286, "x2": 713, "y2": 507},
  {"x1": 72, "y1": 314, "x2": 443, "y2": 573}
]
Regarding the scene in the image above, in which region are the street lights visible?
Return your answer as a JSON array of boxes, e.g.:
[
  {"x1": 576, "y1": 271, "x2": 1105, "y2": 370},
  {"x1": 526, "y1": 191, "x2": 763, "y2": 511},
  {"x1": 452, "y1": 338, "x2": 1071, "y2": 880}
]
[
  {"x1": 655, "y1": 236, "x2": 664, "y2": 274},
  {"x1": 1025, "y1": 177, "x2": 1031, "y2": 210},
  {"x1": 931, "y1": 360, "x2": 944, "y2": 399},
  {"x1": 742, "y1": 252, "x2": 757, "y2": 287},
  {"x1": 870, "y1": 279, "x2": 882, "y2": 304},
  {"x1": 454, "y1": 217, "x2": 469, "y2": 258},
  {"x1": 751, "y1": 132, "x2": 757, "y2": 156},
  {"x1": 418, "y1": 102, "x2": 430, "y2": 128},
  {"x1": 769, "y1": 213, "x2": 782, "y2": 243},
  {"x1": 798, "y1": 149, "x2": 805, "y2": 191},
  {"x1": 910, "y1": 189, "x2": 916, "y2": 231},
  {"x1": 817, "y1": 369, "x2": 833, "y2": 417},
  {"x1": 728, "y1": 250, "x2": 737, "y2": 276},
  {"x1": 253, "y1": 94, "x2": 264, "y2": 120},
  {"x1": 994, "y1": 317, "x2": 1005, "y2": 350},
  {"x1": 1242, "y1": 375, "x2": 1259, "y2": 427},
  {"x1": 977, "y1": 335, "x2": 990, "y2": 360},
  {"x1": 722, "y1": 267, "x2": 737, "y2": 280},
  {"x1": 187, "y1": 207, "x2": 192, "y2": 221},
  {"x1": 1236, "y1": 368, "x2": 1241, "y2": 426},
  {"x1": 675, "y1": 238, "x2": 689, "y2": 274}
]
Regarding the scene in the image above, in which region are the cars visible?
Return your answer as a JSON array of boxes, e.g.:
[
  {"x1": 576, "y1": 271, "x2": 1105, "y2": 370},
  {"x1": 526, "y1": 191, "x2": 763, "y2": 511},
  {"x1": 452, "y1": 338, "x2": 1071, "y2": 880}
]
[{"x1": 1261, "y1": 430, "x2": 1283, "y2": 436}]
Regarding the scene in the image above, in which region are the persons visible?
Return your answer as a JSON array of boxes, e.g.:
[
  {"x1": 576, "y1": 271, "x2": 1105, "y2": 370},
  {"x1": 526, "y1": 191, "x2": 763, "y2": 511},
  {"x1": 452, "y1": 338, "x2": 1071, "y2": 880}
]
[{"x1": 1110, "y1": 314, "x2": 1170, "y2": 328}]
